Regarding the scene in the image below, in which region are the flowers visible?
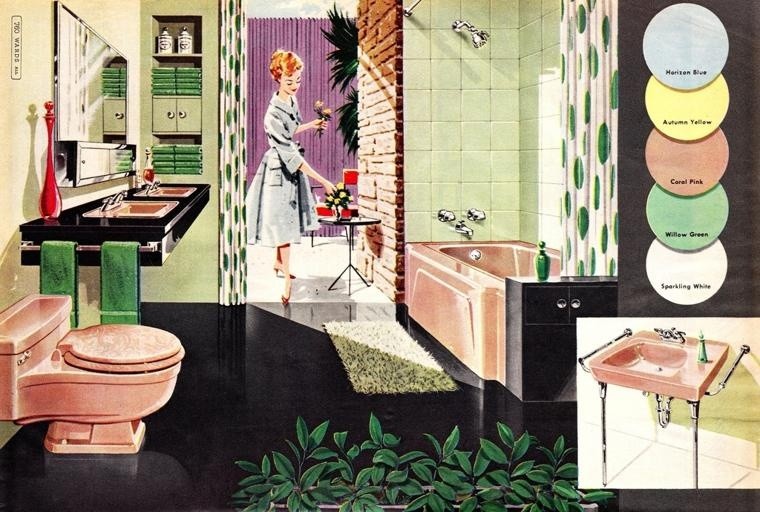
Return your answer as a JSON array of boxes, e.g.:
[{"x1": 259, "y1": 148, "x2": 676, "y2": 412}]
[
  {"x1": 313, "y1": 100, "x2": 333, "y2": 139},
  {"x1": 324, "y1": 182, "x2": 355, "y2": 212}
]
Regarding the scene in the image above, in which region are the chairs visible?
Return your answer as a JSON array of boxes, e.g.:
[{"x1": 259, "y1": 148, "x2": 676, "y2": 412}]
[{"x1": 309, "y1": 168, "x2": 359, "y2": 251}]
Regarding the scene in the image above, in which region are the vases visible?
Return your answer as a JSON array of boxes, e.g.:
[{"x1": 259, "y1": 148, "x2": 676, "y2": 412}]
[
  {"x1": 333, "y1": 210, "x2": 343, "y2": 221},
  {"x1": 38, "y1": 101, "x2": 63, "y2": 225}
]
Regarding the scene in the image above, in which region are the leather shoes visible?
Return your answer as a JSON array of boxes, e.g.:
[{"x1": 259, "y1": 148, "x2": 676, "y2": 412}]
[{"x1": 281, "y1": 282, "x2": 291, "y2": 305}]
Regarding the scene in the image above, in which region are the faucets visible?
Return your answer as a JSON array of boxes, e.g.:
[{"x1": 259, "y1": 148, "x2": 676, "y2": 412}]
[
  {"x1": 103, "y1": 191, "x2": 128, "y2": 207},
  {"x1": 456, "y1": 221, "x2": 474, "y2": 238},
  {"x1": 150, "y1": 180, "x2": 160, "y2": 190},
  {"x1": 654, "y1": 326, "x2": 685, "y2": 343}
]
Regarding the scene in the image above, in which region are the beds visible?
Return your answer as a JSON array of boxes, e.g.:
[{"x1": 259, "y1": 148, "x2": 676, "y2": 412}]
[{"x1": 317, "y1": 217, "x2": 382, "y2": 297}]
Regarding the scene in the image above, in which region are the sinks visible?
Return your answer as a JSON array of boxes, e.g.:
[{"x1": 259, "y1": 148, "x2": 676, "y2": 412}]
[
  {"x1": 589, "y1": 331, "x2": 728, "y2": 402},
  {"x1": 81, "y1": 200, "x2": 179, "y2": 219},
  {"x1": 132, "y1": 187, "x2": 197, "y2": 198}
]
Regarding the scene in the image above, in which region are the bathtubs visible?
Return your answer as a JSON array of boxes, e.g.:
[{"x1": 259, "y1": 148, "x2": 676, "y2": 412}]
[{"x1": 404, "y1": 241, "x2": 562, "y2": 387}]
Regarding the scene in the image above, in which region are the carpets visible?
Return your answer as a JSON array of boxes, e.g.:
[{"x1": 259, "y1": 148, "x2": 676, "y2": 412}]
[{"x1": 322, "y1": 318, "x2": 462, "y2": 396}]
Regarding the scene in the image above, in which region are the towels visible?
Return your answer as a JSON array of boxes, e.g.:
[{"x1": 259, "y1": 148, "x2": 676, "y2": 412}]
[
  {"x1": 99, "y1": 67, "x2": 127, "y2": 101},
  {"x1": 115, "y1": 149, "x2": 133, "y2": 173},
  {"x1": 38, "y1": 239, "x2": 141, "y2": 331},
  {"x1": 151, "y1": 144, "x2": 203, "y2": 176},
  {"x1": 150, "y1": 67, "x2": 202, "y2": 97}
]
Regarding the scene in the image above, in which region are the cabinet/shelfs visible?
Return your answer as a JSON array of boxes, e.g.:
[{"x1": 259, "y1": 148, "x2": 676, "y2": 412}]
[
  {"x1": 59, "y1": 141, "x2": 137, "y2": 188},
  {"x1": 504, "y1": 275, "x2": 620, "y2": 405},
  {"x1": 102, "y1": 99, "x2": 126, "y2": 135},
  {"x1": 149, "y1": 13, "x2": 205, "y2": 178}
]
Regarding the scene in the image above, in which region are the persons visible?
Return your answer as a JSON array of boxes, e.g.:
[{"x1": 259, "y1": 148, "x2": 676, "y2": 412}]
[{"x1": 246, "y1": 49, "x2": 338, "y2": 308}]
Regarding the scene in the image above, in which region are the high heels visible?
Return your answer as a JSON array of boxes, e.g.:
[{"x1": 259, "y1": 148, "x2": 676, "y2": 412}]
[{"x1": 274, "y1": 265, "x2": 296, "y2": 280}]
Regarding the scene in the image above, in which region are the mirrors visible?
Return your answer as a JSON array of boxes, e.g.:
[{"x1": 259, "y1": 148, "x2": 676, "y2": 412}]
[{"x1": 53, "y1": 0, "x2": 129, "y2": 141}]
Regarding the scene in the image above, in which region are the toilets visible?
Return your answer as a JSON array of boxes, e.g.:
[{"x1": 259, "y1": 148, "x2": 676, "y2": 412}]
[{"x1": 0, "y1": 295, "x2": 186, "y2": 455}]
